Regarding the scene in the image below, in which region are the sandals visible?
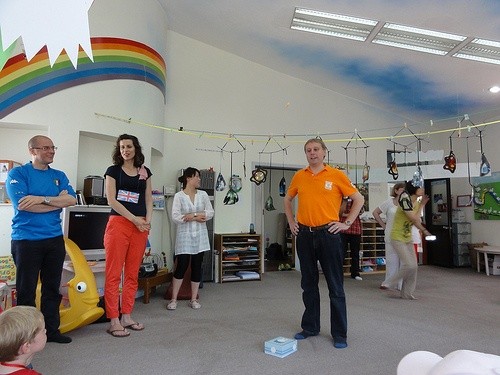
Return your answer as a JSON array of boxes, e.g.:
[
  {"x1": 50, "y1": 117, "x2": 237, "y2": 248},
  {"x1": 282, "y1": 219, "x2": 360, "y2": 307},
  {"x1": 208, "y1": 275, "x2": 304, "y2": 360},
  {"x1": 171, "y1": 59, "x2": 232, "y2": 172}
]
[{"x1": 167, "y1": 299, "x2": 201, "y2": 310}]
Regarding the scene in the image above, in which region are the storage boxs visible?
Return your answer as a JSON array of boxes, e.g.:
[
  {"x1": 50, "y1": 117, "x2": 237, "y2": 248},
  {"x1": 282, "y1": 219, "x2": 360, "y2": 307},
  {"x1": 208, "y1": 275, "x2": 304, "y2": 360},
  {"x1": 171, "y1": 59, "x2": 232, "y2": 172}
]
[{"x1": 451, "y1": 209, "x2": 473, "y2": 267}]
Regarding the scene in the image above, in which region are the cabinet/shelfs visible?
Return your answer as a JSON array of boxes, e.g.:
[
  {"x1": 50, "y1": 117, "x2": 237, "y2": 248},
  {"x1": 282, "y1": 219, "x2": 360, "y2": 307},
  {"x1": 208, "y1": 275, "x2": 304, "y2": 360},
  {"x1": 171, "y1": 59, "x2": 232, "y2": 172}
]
[
  {"x1": 283, "y1": 222, "x2": 295, "y2": 270},
  {"x1": 214, "y1": 233, "x2": 263, "y2": 282},
  {"x1": 343, "y1": 218, "x2": 387, "y2": 277}
]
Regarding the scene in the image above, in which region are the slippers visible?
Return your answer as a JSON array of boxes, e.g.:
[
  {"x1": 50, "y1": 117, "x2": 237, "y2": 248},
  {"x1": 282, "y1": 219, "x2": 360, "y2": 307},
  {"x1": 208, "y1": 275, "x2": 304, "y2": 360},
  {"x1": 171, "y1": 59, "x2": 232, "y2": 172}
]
[{"x1": 106, "y1": 322, "x2": 145, "y2": 337}]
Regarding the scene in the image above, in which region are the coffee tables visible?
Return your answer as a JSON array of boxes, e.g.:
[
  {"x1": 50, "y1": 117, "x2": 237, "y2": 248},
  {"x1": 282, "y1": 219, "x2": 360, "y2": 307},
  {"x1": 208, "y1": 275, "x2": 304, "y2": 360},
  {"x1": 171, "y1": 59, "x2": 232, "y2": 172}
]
[{"x1": 137, "y1": 271, "x2": 173, "y2": 304}]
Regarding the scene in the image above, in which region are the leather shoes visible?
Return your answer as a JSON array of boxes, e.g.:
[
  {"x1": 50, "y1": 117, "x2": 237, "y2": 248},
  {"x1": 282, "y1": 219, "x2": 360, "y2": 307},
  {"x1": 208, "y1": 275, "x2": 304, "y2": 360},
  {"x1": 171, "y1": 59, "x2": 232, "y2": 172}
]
[{"x1": 46, "y1": 333, "x2": 72, "y2": 343}]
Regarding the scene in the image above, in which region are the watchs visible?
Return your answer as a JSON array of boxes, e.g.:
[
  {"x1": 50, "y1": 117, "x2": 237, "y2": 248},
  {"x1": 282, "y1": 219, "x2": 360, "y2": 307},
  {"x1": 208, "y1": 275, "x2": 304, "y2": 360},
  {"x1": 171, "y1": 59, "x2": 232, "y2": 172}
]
[{"x1": 42, "y1": 196, "x2": 51, "y2": 205}]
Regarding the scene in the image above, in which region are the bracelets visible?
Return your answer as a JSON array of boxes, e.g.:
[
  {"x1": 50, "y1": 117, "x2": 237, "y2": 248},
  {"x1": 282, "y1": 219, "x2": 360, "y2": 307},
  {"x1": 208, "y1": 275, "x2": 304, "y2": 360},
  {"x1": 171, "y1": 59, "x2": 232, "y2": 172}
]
[{"x1": 193, "y1": 212, "x2": 197, "y2": 217}]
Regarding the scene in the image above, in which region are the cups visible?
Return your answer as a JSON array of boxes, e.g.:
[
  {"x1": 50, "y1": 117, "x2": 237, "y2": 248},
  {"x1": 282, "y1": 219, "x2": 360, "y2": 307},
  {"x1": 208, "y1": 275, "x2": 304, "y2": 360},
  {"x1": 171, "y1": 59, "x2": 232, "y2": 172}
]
[{"x1": 250, "y1": 223, "x2": 254, "y2": 233}]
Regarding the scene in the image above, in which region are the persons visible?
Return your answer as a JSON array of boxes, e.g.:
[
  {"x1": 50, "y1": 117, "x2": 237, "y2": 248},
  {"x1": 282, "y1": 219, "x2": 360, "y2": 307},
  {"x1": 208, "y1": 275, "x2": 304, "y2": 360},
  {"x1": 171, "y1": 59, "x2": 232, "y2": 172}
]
[
  {"x1": 338, "y1": 186, "x2": 362, "y2": 281},
  {"x1": 381, "y1": 179, "x2": 432, "y2": 299},
  {"x1": 4, "y1": 134, "x2": 75, "y2": 345},
  {"x1": 283, "y1": 139, "x2": 364, "y2": 349},
  {"x1": 0, "y1": 306, "x2": 49, "y2": 375},
  {"x1": 372, "y1": 181, "x2": 403, "y2": 291},
  {"x1": 102, "y1": 133, "x2": 154, "y2": 336},
  {"x1": 166, "y1": 166, "x2": 214, "y2": 311}
]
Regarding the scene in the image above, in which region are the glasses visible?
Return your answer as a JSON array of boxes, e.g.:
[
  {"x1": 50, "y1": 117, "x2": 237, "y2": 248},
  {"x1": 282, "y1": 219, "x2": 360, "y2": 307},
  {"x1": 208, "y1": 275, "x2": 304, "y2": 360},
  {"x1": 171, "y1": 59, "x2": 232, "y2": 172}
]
[{"x1": 32, "y1": 146, "x2": 57, "y2": 151}]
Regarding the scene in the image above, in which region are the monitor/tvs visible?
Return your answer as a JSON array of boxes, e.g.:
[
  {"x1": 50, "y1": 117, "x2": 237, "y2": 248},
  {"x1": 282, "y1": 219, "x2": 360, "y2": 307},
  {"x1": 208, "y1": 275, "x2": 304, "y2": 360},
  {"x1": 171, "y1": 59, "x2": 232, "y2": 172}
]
[{"x1": 64, "y1": 207, "x2": 128, "y2": 261}]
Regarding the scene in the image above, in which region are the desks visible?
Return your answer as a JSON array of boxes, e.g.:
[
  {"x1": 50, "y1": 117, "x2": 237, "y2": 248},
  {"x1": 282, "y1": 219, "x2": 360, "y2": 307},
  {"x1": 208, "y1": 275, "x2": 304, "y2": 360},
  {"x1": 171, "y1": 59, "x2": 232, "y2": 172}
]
[{"x1": 474, "y1": 246, "x2": 500, "y2": 277}]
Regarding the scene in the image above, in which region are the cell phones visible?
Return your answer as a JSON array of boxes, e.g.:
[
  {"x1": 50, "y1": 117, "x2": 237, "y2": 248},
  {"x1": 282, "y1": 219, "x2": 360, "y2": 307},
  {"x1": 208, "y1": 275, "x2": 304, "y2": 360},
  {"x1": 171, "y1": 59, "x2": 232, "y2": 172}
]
[{"x1": 425, "y1": 235, "x2": 436, "y2": 241}]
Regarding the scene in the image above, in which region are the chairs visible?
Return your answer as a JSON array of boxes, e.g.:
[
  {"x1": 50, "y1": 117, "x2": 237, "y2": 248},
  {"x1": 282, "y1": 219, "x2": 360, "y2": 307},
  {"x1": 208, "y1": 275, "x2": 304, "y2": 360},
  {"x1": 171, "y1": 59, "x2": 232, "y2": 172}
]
[{"x1": 466, "y1": 242, "x2": 490, "y2": 272}]
